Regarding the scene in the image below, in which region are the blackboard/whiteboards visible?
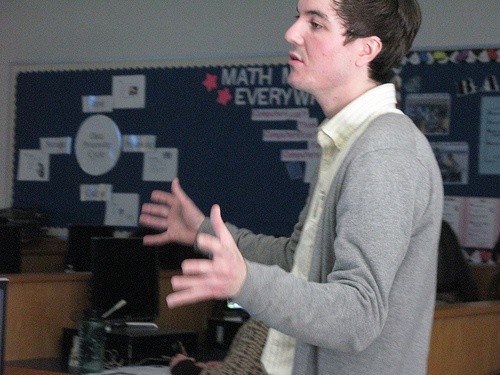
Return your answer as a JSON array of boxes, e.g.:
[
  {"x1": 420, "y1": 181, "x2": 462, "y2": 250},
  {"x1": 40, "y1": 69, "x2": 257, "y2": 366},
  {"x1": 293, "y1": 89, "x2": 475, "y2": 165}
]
[{"x1": 4, "y1": 42, "x2": 500, "y2": 264}]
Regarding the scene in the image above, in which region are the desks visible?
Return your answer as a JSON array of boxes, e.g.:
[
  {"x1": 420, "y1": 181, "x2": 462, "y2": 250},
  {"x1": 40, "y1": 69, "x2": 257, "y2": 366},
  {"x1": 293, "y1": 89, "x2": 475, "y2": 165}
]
[
  {"x1": 426, "y1": 301, "x2": 500, "y2": 375},
  {"x1": 4, "y1": 344, "x2": 229, "y2": 374},
  {"x1": 1, "y1": 268, "x2": 213, "y2": 362}
]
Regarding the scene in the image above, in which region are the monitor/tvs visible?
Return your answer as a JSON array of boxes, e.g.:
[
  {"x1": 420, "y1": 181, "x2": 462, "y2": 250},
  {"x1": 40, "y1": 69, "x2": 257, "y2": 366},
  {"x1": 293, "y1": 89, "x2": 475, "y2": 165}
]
[
  {"x1": 66, "y1": 224, "x2": 160, "y2": 272},
  {"x1": 92, "y1": 237, "x2": 160, "y2": 318},
  {"x1": 225, "y1": 298, "x2": 248, "y2": 318},
  {"x1": 0, "y1": 224, "x2": 22, "y2": 274}
]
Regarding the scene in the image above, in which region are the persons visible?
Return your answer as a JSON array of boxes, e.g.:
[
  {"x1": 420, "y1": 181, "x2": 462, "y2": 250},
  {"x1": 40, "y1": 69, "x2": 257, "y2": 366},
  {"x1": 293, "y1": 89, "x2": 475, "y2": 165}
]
[
  {"x1": 139, "y1": 0, "x2": 445, "y2": 375},
  {"x1": 436, "y1": 215, "x2": 499, "y2": 304},
  {"x1": 168, "y1": 352, "x2": 224, "y2": 375}
]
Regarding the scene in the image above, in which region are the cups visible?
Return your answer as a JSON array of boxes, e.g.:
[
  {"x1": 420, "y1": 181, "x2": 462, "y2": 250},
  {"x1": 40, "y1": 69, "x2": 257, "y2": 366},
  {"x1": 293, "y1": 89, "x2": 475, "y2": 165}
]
[{"x1": 80, "y1": 317, "x2": 104, "y2": 373}]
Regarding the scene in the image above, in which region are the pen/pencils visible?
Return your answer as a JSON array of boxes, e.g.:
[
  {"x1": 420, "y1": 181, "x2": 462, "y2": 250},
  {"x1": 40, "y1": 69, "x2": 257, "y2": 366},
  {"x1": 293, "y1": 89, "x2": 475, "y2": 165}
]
[
  {"x1": 100, "y1": 299, "x2": 127, "y2": 319},
  {"x1": 162, "y1": 355, "x2": 172, "y2": 359},
  {"x1": 150, "y1": 357, "x2": 170, "y2": 361},
  {"x1": 176, "y1": 340, "x2": 187, "y2": 356}
]
